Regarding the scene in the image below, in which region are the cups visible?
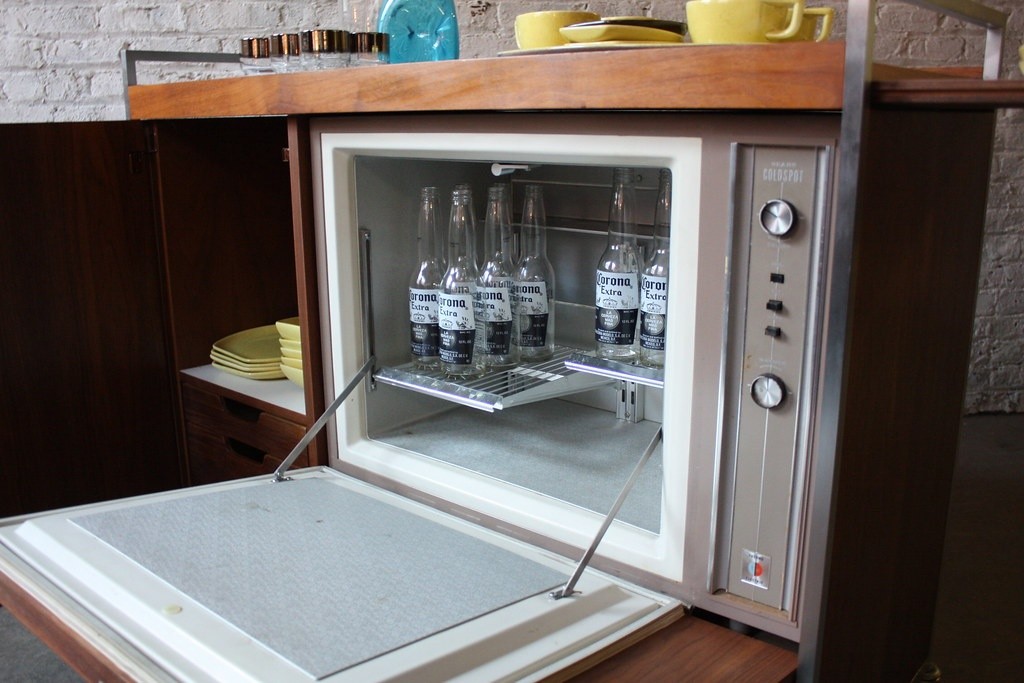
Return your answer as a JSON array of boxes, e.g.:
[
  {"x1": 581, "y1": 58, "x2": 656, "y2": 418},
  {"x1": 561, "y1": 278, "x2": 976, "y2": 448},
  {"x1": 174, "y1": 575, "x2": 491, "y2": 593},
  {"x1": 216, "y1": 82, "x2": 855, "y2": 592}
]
[
  {"x1": 686, "y1": 0, "x2": 804, "y2": 43},
  {"x1": 785, "y1": 8, "x2": 835, "y2": 43},
  {"x1": 514, "y1": 11, "x2": 601, "y2": 49}
]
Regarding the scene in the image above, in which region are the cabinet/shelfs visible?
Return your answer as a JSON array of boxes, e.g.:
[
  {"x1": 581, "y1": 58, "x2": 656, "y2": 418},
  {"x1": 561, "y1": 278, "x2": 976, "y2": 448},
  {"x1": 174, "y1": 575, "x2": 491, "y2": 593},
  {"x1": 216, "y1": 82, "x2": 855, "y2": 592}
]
[{"x1": 119, "y1": 0, "x2": 1010, "y2": 681}]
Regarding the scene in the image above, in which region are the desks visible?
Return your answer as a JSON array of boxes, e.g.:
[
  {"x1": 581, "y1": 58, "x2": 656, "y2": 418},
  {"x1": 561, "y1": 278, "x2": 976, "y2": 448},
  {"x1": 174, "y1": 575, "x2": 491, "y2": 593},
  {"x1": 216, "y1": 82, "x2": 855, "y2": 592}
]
[{"x1": 0, "y1": 456, "x2": 805, "y2": 683}]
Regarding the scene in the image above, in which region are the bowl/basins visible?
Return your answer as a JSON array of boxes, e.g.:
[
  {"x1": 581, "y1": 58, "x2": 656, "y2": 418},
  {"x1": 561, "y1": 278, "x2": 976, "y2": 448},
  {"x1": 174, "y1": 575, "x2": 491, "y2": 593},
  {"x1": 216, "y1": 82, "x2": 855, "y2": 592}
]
[{"x1": 276, "y1": 316, "x2": 304, "y2": 388}]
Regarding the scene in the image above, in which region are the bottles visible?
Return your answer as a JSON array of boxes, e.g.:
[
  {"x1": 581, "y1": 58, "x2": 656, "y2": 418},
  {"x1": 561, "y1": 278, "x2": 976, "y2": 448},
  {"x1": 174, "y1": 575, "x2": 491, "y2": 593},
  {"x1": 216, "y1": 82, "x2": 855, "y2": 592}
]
[
  {"x1": 478, "y1": 184, "x2": 515, "y2": 367},
  {"x1": 516, "y1": 185, "x2": 556, "y2": 363},
  {"x1": 411, "y1": 186, "x2": 447, "y2": 367},
  {"x1": 439, "y1": 184, "x2": 479, "y2": 375},
  {"x1": 594, "y1": 167, "x2": 641, "y2": 360},
  {"x1": 641, "y1": 169, "x2": 672, "y2": 368}
]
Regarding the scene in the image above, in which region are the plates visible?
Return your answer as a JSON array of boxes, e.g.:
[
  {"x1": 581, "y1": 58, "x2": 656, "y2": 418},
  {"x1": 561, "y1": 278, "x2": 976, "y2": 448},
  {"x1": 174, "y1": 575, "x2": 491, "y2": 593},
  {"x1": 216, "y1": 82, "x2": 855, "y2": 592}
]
[
  {"x1": 210, "y1": 325, "x2": 286, "y2": 380},
  {"x1": 560, "y1": 25, "x2": 684, "y2": 42},
  {"x1": 561, "y1": 19, "x2": 688, "y2": 35}
]
